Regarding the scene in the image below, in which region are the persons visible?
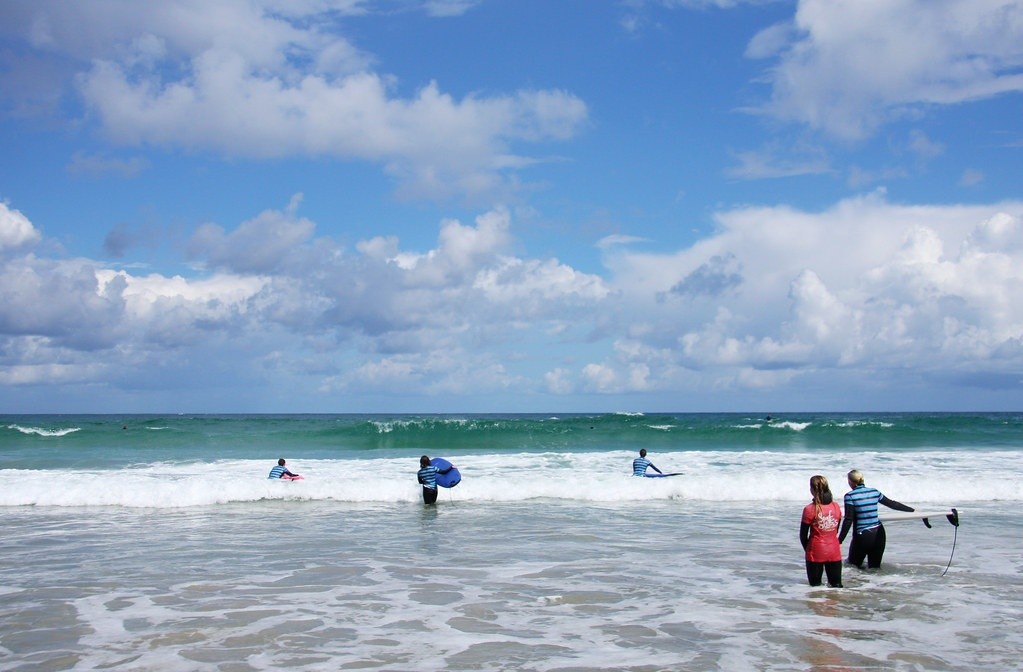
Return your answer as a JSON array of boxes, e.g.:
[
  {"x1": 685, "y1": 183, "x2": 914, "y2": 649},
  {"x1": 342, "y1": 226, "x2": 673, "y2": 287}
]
[
  {"x1": 800, "y1": 475, "x2": 843, "y2": 588},
  {"x1": 268, "y1": 459, "x2": 299, "y2": 479},
  {"x1": 838, "y1": 470, "x2": 915, "y2": 570},
  {"x1": 633, "y1": 449, "x2": 662, "y2": 475},
  {"x1": 418, "y1": 456, "x2": 457, "y2": 503}
]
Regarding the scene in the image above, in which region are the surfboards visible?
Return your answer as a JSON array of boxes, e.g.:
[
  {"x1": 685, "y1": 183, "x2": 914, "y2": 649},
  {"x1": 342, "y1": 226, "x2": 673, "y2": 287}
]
[
  {"x1": 283, "y1": 475, "x2": 304, "y2": 480},
  {"x1": 646, "y1": 473, "x2": 685, "y2": 478},
  {"x1": 430, "y1": 457, "x2": 461, "y2": 489},
  {"x1": 842, "y1": 510, "x2": 964, "y2": 522}
]
